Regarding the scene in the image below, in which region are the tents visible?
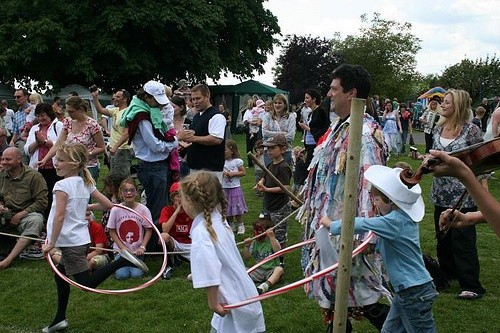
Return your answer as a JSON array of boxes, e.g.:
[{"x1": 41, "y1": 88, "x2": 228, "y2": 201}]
[
  {"x1": 209, "y1": 79, "x2": 288, "y2": 134},
  {"x1": 0, "y1": 85, "x2": 21, "y2": 108},
  {"x1": 37, "y1": 84, "x2": 113, "y2": 120}
]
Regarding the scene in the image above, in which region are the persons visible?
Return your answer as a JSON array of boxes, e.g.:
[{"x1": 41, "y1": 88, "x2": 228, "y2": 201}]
[{"x1": 0, "y1": 64, "x2": 500, "y2": 333}]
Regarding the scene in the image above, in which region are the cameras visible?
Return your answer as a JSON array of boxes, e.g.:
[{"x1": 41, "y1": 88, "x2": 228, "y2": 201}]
[{"x1": 182, "y1": 125, "x2": 190, "y2": 130}]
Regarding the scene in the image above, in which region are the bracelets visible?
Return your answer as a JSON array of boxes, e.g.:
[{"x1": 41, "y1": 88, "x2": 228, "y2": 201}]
[
  {"x1": 90, "y1": 151, "x2": 92, "y2": 156},
  {"x1": 44, "y1": 140, "x2": 47, "y2": 145}
]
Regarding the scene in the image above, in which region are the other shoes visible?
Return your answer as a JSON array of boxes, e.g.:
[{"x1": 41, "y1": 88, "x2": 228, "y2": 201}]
[
  {"x1": 120, "y1": 249, "x2": 149, "y2": 272},
  {"x1": 256, "y1": 283, "x2": 269, "y2": 294},
  {"x1": 163, "y1": 266, "x2": 173, "y2": 279},
  {"x1": 231, "y1": 226, "x2": 235, "y2": 232},
  {"x1": 41, "y1": 319, "x2": 69, "y2": 333},
  {"x1": 236, "y1": 225, "x2": 245, "y2": 235},
  {"x1": 187, "y1": 273, "x2": 192, "y2": 281},
  {"x1": 459, "y1": 291, "x2": 481, "y2": 299}
]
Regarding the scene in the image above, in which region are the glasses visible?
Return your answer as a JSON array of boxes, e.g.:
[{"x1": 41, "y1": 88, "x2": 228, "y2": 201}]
[
  {"x1": 122, "y1": 88, "x2": 128, "y2": 98},
  {"x1": 13, "y1": 96, "x2": 25, "y2": 99},
  {"x1": 112, "y1": 98, "x2": 116, "y2": 100},
  {"x1": 121, "y1": 188, "x2": 135, "y2": 193},
  {"x1": 66, "y1": 108, "x2": 80, "y2": 114}
]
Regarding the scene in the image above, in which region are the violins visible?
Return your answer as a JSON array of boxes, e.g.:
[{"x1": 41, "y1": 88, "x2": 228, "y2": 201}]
[{"x1": 399, "y1": 136, "x2": 500, "y2": 189}]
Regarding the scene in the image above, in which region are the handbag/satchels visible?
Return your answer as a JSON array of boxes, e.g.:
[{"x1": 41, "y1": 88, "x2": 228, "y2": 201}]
[
  {"x1": 423, "y1": 254, "x2": 449, "y2": 291},
  {"x1": 410, "y1": 134, "x2": 415, "y2": 146}
]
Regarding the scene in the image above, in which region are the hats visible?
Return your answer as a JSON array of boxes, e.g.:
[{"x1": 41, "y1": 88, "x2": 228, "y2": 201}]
[
  {"x1": 142, "y1": 80, "x2": 170, "y2": 105},
  {"x1": 170, "y1": 182, "x2": 180, "y2": 194},
  {"x1": 179, "y1": 79, "x2": 191, "y2": 89},
  {"x1": 260, "y1": 134, "x2": 288, "y2": 147},
  {"x1": 364, "y1": 164, "x2": 425, "y2": 223},
  {"x1": 256, "y1": 99, "x2": 265, "y2": 107}
]
